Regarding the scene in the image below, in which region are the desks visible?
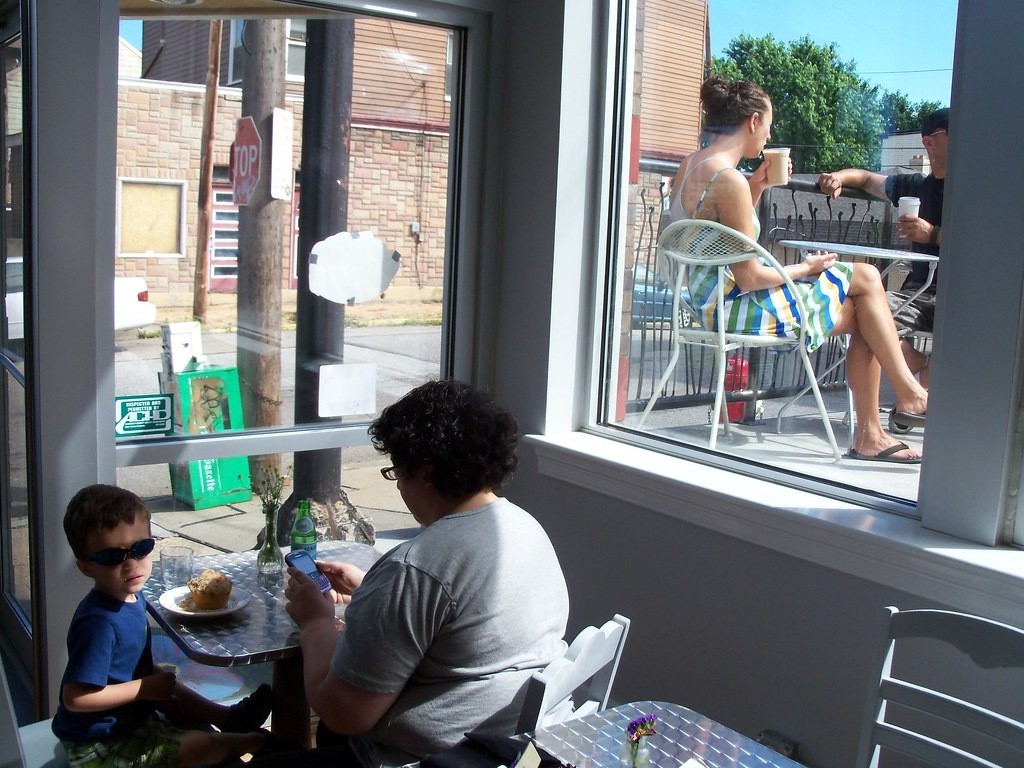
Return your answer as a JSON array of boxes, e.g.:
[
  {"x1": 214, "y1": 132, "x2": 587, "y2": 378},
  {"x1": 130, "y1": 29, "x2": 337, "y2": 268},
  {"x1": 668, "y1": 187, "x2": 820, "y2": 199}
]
[
  {"x1": 777, "y1": 240, "x2": 940, "y2": 456},
  {"x1": 502, "y1": 700, "x2": 808, "y2": 768},
  {"x1": 135, "y1": 541, "x2": 388, "y2": 768}
]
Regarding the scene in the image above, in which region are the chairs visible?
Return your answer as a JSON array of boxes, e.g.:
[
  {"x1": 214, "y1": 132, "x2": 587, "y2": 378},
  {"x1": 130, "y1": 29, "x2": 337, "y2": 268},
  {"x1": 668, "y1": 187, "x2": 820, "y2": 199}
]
[
  {"x1": 333, "y1": 614, "x2": 635, "y2": 768},
  {"x1": 0, "y1": 651, "x2": 87, "y2": 768},
  {"x1": 633, "y1": 220, "x2": 845, "y2": 461},
  {"x1": 855, "y1": 600, "x2": 1024, "y2": 767}
]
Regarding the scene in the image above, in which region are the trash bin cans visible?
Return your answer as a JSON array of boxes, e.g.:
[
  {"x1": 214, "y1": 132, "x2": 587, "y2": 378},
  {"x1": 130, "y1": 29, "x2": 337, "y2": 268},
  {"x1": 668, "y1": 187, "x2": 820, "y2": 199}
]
[{"x1": 159, "y1": 366, "x2": 251, "y2": 511}]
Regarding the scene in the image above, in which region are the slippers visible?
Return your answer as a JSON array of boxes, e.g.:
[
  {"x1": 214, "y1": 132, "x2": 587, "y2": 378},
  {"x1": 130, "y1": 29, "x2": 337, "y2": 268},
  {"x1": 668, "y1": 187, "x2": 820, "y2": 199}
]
[
  {"x1": 849, "y1": 437, "x2": 923, "y2": 464},
  {"x1": 891, "y1": 405, "x2": 927, "y2": 428}
]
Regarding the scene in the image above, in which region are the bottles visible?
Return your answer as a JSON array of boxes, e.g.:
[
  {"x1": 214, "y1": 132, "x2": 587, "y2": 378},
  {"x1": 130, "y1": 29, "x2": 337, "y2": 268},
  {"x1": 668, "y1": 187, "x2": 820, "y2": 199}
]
[{"x1": 290, "y1": 500, "x2": 316, "y2": 561}]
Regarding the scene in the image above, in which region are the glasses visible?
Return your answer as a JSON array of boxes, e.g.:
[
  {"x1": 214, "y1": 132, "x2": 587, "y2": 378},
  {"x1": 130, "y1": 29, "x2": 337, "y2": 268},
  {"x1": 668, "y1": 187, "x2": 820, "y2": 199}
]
[
  {"x1": 380, "y1": 464, "x2": 406, "y2": 480},
  {"x1": 85, "y1": 537, "x2": 157, "y2": 568}
]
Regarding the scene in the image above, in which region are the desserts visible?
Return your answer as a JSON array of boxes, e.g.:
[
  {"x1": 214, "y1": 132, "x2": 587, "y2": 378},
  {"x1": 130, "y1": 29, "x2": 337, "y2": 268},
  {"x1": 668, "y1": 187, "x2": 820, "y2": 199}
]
[{"x1": 188, "y1": 568, "x2": 233, "y2": 610}]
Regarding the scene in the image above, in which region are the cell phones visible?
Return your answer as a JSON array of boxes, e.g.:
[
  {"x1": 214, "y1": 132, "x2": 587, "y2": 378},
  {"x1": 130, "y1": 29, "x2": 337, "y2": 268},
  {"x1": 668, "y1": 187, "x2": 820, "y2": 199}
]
[{"x1": 285, "y1": 549, "x2": 332, "y2": 594}]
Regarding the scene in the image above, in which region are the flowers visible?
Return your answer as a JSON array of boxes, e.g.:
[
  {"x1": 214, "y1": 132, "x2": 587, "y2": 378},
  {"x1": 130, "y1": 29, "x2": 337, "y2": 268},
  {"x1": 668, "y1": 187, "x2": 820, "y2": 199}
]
[
  {"x1": 230, "y1": 462, "x2": 295, "y2": 508},
  {"x1": 626, "y1": 715, "x2": 660, "y2": 743}
]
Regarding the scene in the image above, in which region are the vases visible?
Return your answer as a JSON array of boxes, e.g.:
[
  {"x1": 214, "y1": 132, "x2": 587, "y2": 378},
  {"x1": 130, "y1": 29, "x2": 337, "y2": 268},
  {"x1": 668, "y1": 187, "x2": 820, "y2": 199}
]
[
  {"x1": 258, "y1": 500, "x2": 282, "y2": 577},
  {"x1": 617, "y1": 733, "x2": 649, "y2": 768}
]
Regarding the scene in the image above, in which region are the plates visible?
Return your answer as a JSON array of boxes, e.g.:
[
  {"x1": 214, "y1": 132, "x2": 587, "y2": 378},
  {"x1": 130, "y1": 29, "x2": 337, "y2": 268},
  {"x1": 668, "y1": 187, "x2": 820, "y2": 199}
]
[{"x1": 158, "y1": 585, "x2": 251, "y2": 615}]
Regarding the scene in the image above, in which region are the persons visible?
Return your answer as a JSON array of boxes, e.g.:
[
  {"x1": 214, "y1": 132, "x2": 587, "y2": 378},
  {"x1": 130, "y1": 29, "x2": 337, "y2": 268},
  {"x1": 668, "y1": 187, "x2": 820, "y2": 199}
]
[
  {"x1": 51, "y1": 484, "x2": 277, "y2": 768},
  {"x1": 659, "y1": 75, "x2": 927, "y2": 464},
  {"x1": 286, "y1": 379, "x2": 569, "y2": 768},
  {"x1": 818, "y1": 106, "x2": 950, "y2": 429}
]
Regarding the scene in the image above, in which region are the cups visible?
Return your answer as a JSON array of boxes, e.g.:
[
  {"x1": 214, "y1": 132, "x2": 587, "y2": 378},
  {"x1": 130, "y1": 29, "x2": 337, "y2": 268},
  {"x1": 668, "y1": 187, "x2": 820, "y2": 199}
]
[
  {"x1": 898, "y1": 197, "x2": 921, "y2": 237},
  {"x1": 762, "y1": 147, "x2": 792, "y2": 186},
  {"x1": 160, "y1": 546, "x2": 194, "y2": 592}
]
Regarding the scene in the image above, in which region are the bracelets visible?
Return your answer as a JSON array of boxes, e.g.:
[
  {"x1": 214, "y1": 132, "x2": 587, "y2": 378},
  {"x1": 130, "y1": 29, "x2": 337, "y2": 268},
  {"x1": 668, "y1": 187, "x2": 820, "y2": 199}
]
[{"x1": 930, "y1": 225, "x2": 940, "y2": 248}]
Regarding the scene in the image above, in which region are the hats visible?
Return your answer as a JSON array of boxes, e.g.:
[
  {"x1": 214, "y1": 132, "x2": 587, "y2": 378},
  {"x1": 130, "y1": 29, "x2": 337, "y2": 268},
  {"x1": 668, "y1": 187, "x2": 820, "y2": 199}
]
[{"x1": 921, "y1": 108, "x2": 950, "y2": 137}]
